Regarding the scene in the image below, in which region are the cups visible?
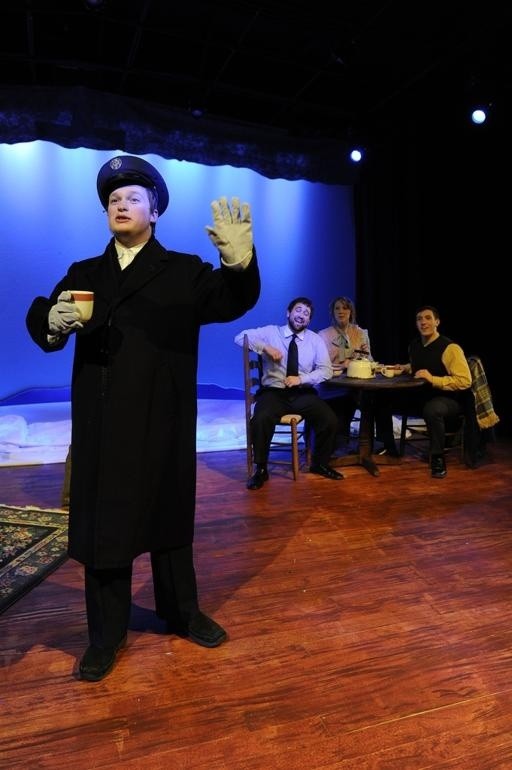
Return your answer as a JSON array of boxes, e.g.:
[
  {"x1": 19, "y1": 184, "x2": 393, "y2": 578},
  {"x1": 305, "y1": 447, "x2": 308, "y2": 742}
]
[
  {"x1": 381, "y1": 365, "x2": 395, "y2": 377},
  {"x1": 332, "y1": 364, "x2": 344, "y2": 377},
  {"x1": 394, "y1": 367, "x2": 403, "y2": 376},
  {"x1": 67, "y1": 291, "x2": 94, "y2": 324}
]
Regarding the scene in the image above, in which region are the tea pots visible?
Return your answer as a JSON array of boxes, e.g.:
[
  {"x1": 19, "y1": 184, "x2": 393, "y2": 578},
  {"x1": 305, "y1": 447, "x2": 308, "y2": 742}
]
[{"x1": 347, "y1": 349, "x2": 376, "y2": 379}]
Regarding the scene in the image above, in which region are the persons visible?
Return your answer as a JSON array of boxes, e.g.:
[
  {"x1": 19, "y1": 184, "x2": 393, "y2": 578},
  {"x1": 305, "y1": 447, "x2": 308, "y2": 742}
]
[
  {"x1": 318, "y1": 297, "x2": 381, "y2": 478},
  {"x1": 373, "y1": 307, "x2": 474, "y2": 479},
  {"x1": 25, "y1": 156, "x2": 261, "y2": 682},
  {"x1": 233, "y1": 296, "x2": 344, "y2": 491}
]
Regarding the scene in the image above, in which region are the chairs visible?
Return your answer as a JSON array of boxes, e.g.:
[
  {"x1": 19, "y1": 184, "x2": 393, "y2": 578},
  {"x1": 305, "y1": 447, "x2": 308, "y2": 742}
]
[
  {"x1": 243, "y1": 332, "x2": 313, "y2": 482},
  {"x1": 399, "y1": 357, "x2": 482, "y2": 465}
]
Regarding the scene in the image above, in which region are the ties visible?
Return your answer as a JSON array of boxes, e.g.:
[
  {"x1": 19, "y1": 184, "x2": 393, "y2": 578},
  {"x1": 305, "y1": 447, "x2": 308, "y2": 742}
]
[{"x1": 287, "y1": 335, "x2": 299, "y2": 390}]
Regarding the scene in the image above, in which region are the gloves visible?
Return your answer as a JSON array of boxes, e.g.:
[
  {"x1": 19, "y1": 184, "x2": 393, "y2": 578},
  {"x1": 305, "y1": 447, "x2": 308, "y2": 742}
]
[
  {"x1": 204, "y1": 196, "x2": 256, "y2": 274},
  {"x1": 44, "y1": 291, "x2": 87, "y2": 343}
]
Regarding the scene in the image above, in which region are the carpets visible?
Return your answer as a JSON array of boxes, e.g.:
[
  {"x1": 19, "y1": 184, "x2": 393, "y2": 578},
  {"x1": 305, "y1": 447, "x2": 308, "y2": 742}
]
[{"x1": 0, "y1": 503, "x2": 71, "y2": 614}]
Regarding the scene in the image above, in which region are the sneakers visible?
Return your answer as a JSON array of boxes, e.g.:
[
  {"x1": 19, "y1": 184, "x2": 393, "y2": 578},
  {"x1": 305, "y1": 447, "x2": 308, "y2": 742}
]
[{"x1": 361, "y1": 458, "x2": 380, "y2": 479}]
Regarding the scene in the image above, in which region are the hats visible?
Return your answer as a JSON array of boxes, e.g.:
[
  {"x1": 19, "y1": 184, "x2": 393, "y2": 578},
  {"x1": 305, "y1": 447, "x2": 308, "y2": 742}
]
[{"x1": 96, "y1": 155, "x2": 170, "y2": 220}]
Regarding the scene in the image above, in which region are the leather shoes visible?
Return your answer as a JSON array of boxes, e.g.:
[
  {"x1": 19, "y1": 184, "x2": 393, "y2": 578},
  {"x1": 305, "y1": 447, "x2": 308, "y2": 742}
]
[
  {"x1": 156, "y1": 606, "x2": 228, "y2": 650},
  {"x1": 308, "y1": 464, "x2": 343, "y2": 480},
  {"x1": 75, "y1": 630, "x2": 129, "y2": 681},
  {"x1": 431, "y1": 456, "x2": 446, "y2": 478},
  {"x1": 376, "y1": 444, "x2": 399, "y2": 457},
  {"x1": 246, "y1": 468, "x2": 270, "y2": 490}
]
